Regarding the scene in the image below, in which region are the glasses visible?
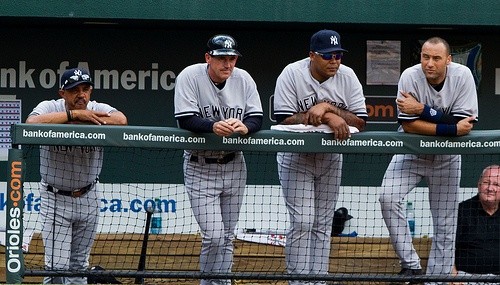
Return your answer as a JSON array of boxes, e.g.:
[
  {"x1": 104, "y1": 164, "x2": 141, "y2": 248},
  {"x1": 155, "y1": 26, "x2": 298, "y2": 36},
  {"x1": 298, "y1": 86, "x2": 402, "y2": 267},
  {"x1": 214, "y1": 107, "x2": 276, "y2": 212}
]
[{"x1": 314, "y1": 51, "x2": 343, "y2": 60}]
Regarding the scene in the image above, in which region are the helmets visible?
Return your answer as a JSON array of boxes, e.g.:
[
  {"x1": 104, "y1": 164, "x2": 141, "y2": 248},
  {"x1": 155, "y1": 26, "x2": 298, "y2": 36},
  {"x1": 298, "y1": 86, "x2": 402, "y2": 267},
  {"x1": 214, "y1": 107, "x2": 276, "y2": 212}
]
[{"x1": 206, "y1": 35, "x2": 243, "y2": 57}]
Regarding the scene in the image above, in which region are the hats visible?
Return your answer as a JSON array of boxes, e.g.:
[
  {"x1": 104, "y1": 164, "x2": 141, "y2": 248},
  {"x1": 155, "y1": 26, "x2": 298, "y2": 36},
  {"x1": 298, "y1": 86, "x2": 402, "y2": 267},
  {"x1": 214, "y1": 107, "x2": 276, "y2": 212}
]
[
  {"x1": 60, "y1": 67, "x2": 95, "y2": 90},
  {"x1": 310, "y1": 29, "x2": 349, "y2": 54}
]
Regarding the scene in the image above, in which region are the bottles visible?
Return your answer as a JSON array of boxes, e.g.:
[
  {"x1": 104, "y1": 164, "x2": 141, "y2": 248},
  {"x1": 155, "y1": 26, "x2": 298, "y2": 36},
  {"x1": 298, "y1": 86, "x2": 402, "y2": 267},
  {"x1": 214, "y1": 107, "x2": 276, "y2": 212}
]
[
  {"x1": 405, "y1": 202, "x2": 415, "y2": 237},
  {"x1": 150, "y1": 198, "x2": 162, "y2": 235}
]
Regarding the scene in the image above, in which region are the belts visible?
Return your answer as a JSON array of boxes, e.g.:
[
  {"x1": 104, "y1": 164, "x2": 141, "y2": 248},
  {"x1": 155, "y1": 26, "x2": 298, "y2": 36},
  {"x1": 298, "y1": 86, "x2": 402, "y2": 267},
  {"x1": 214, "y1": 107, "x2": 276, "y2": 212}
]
[
  {"x1": 191, "y1": 151, "x2": 236, "y2": 165},
  {"x1": 46, "y1": 178, "x2": 98, "y2": 198}
]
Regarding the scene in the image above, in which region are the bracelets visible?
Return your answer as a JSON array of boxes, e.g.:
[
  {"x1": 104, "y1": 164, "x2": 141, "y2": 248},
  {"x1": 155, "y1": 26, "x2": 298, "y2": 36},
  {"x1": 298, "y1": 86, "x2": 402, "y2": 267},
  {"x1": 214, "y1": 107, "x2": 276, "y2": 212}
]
[
  {"x1": 70, "y1": 109, "x2": 74, "y2": 120},
  {"x1": 67, "y1": 108, "x2": 71, "y2": 121}
]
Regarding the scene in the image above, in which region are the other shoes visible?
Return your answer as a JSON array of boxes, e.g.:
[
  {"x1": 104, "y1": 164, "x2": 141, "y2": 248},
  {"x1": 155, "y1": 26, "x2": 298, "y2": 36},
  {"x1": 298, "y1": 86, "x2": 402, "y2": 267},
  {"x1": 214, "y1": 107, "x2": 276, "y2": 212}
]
[{"x1": 200, "y1": 279, "x2": 232, "y2": 285}]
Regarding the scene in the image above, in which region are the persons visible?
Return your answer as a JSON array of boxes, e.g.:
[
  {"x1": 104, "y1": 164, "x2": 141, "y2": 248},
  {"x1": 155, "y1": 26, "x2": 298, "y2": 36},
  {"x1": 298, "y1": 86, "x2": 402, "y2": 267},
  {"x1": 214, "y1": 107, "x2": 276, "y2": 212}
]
[
  {"x1": 174, "y1": 35, "x2": 263, "y2": 285},
  {"x1": 274, "y1": 30, "x2": 368, "y2": 285},
  {"x1": 26, "y1": 69, "x2": 128, "y2": 285},
  {"x1": 379, "y1": 38, "x2": 479, "y2": 285},
  {"x1": 449, "y1": 164, "x2": 500, "y2": 285}
]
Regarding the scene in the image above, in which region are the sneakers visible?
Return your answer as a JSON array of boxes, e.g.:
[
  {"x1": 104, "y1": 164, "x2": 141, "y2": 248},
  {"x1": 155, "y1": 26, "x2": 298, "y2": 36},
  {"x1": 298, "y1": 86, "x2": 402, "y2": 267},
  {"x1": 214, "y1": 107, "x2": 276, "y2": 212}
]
[{"x1": 391, "y1": 268, "x2": 422, "y2": 285}]
